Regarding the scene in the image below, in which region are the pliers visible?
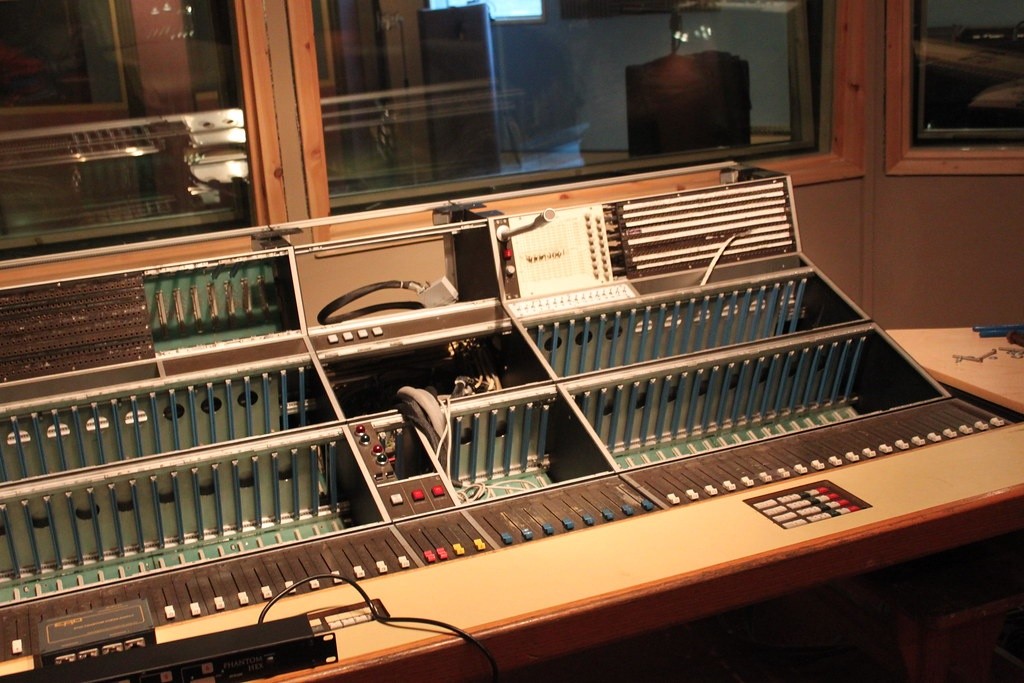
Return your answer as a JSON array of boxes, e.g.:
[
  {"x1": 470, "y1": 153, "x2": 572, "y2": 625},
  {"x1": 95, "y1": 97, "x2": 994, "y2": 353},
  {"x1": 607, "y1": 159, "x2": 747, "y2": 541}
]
[{"x1": 972, "y1": 324, "x2": 1024, "y2": 337}]
